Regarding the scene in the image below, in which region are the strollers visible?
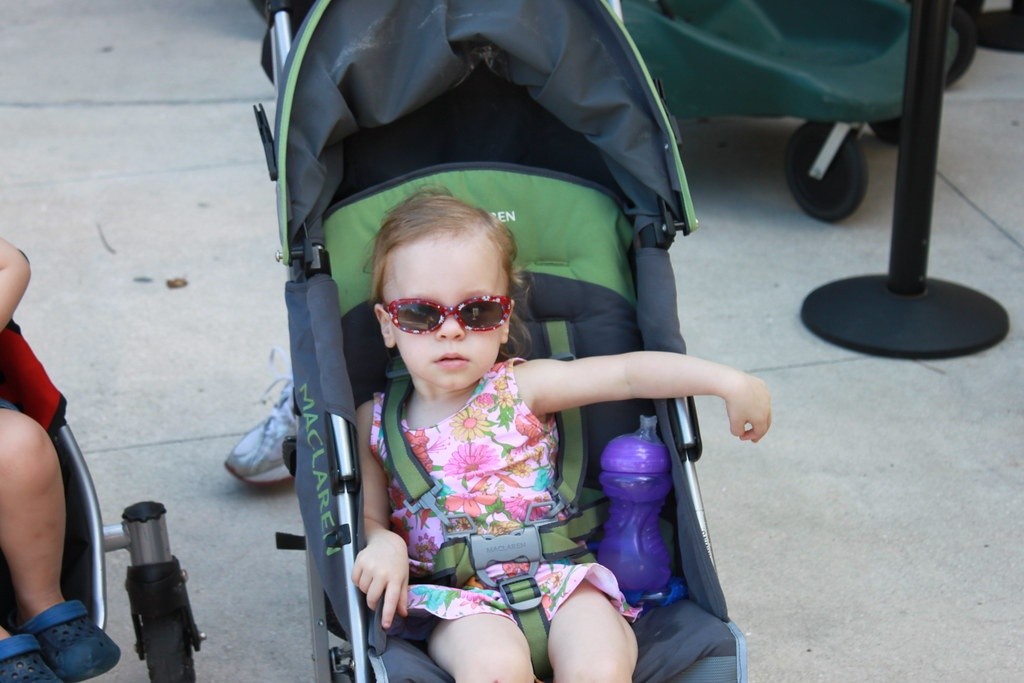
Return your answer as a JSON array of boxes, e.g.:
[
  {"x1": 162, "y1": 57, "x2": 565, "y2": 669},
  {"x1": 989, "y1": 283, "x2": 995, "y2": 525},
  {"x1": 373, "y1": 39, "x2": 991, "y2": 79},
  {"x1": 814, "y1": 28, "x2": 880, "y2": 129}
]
[
  {"x1": 252, "y1": 0, "x2": 747, "y2": 683},
  {"x1": 0, "y1": 318, "x2": 208, "y2": 683}
]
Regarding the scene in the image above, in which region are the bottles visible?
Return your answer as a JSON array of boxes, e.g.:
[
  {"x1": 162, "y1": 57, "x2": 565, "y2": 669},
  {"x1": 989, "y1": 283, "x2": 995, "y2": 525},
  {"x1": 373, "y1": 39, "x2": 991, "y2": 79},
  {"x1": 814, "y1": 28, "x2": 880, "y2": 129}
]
[{"x1": 600, "y1": 414, "x2": 678, "y2": 594}]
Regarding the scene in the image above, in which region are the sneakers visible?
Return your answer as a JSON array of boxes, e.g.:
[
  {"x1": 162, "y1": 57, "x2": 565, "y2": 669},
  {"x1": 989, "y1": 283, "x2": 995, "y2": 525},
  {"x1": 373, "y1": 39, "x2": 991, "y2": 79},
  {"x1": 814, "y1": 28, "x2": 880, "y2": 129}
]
[{"x1": 225, "y1": 347, "x2": 300, "y2": 485}]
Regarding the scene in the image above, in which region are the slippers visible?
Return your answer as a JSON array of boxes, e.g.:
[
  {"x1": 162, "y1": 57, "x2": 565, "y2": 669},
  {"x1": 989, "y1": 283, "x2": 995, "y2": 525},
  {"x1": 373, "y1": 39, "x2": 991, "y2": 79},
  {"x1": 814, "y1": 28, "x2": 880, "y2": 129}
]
[
  {"x1": 0, "y1": 633, "x2": 64, "y2": 683},
  {"x1": 7, "y1": 589, "x2": 121, "y2": 683}
]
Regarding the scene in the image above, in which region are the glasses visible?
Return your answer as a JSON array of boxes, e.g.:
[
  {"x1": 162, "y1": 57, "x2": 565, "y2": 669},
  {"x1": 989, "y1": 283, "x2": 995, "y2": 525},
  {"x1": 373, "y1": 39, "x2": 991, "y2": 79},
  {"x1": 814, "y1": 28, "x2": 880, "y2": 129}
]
[{"x1": 381, "y1": 295, "x2": 511, "y2": 335}]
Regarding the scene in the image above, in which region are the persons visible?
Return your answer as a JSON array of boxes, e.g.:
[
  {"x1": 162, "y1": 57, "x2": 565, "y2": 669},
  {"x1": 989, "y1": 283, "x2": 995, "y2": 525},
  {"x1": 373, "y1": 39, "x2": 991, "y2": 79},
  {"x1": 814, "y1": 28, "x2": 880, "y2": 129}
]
[
  {"x1": 0, "y1": 237, "x2": 121, "y2": 683},
  {"x1": 225, "y1": 374, "x2": 298, "y2": 483},
  {"x1": 349, "y1": 192, "x2": 771, "y2": 681}
]
